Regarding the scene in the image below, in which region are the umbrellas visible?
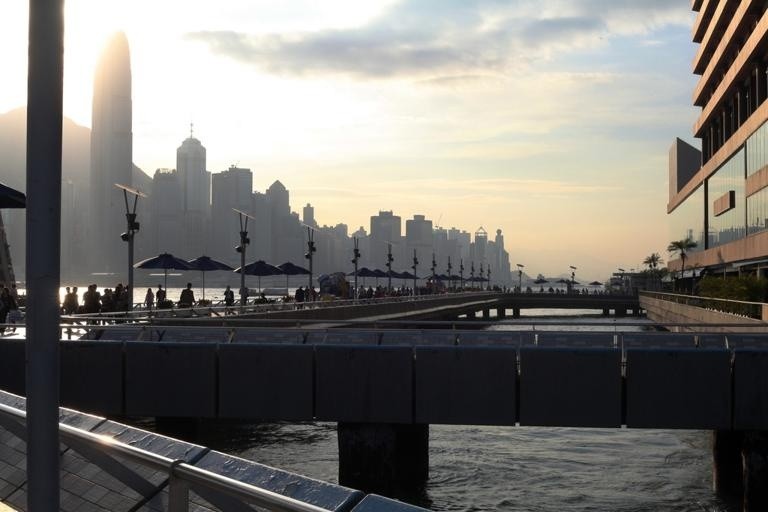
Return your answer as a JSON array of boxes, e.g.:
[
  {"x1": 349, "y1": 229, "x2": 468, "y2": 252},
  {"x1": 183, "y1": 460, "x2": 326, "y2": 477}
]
[{"x1": 533, "y1": 278, "x2": 602, "y2": 292}]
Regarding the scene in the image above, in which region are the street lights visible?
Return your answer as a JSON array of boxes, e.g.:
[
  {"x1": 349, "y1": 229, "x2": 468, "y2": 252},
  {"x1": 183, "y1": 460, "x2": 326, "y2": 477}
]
[
  {"x1": 384, "y1": 239, "x2": 396, "y2": 296},
  {"x1": 231, "y1": 205, "x2": 256, "y2": 314},
  {"x1": 569, "y1": 265, "x2": 578, "y2": 286},
  {"x1": 515, "y1": 263, "x2": 525, "y2": 291},
  {"x1": 114, "y1": 181, "x2": 148, "y2": 314},
  {"x1": 299, "y1": 220, "x2": 321, "y2": 306},
  {"x1": 446, "y1": 255, "x2": 453, "y2": 293},
  {"x1": 618, "y1": 267, "x2": 625, "y2": 288},
  {"x1": 350, "y1": 235, "x2": 363, "y2": 299},
  {"x1": 409, "y1": 247, "x2": 418, "y2": 295},
  {"x1": 457, "y1": 258, "x2": 492, "y2": 292},
  {"x1": 429, "y1": 251, "x2": 438, "y2": 293}
]
